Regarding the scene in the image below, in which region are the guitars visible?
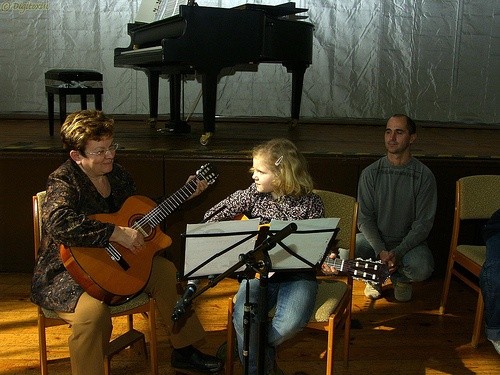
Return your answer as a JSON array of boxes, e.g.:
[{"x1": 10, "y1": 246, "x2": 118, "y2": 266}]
[
  {"x1": 323, "y1": 254, "x2": 386, "y2": 284},
  {"x1": 60, "y1": 161, "x2": 221, "y2": 308}
]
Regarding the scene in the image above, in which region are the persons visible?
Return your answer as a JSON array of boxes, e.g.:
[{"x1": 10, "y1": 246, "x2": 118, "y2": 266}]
[
  {"x1": 202, "y1": 138, "x2": 341, "y2": 374},
  {"x1": 31, "y1": 110, "x2": 226, "y2": 375},
  {"x1": 338, "y1": 113, "x2": 438, "y2": 302}
]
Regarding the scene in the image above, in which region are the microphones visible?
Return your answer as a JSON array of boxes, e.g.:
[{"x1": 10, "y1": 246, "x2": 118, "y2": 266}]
[{"x1": 172, "y1": 278, "x2": 200, "y2": 320}]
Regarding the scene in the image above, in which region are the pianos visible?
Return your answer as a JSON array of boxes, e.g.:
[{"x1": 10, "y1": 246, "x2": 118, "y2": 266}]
[{"x1": 114, "y1": 0, "x2": 315, "y2": 148}]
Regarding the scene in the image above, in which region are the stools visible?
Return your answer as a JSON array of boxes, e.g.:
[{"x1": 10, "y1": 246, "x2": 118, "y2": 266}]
[{"x1": 45, "y1": 69, "x2": 103, "y2": 137}]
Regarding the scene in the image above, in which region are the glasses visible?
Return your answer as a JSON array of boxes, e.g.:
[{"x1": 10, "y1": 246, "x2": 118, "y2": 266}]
[{"x1": 84, "y1": 143, "x2": 119, "y2": 157}]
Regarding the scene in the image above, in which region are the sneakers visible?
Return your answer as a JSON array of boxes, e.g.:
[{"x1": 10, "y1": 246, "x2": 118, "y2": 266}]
[
  {"x1": 364, "y1": 282, "x2": 383, "y2": 299},
  {"x1": 170, "y1": 344, "x2": 223, "y2": 375},
  {"x1": 485, "y1": 329, "x2": 499, "y2": 352},
  {"x1": 393, "y1": 281, "x2": 413, "y2": 301},
  {"x1": 216, "y1": 336, "x2": 239, "y2": 360}
]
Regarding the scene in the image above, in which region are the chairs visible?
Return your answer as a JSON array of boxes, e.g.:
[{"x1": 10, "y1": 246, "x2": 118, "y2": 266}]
[
  {"x1": 33, "y1": 191, "x2": 158, "y2": 375},
  {"x1": 226, "y1": 189, "x2": 359, "y2": 375},
  {"x1": 438, "y1": 175, "x2": 500, "y2": 348}
]
